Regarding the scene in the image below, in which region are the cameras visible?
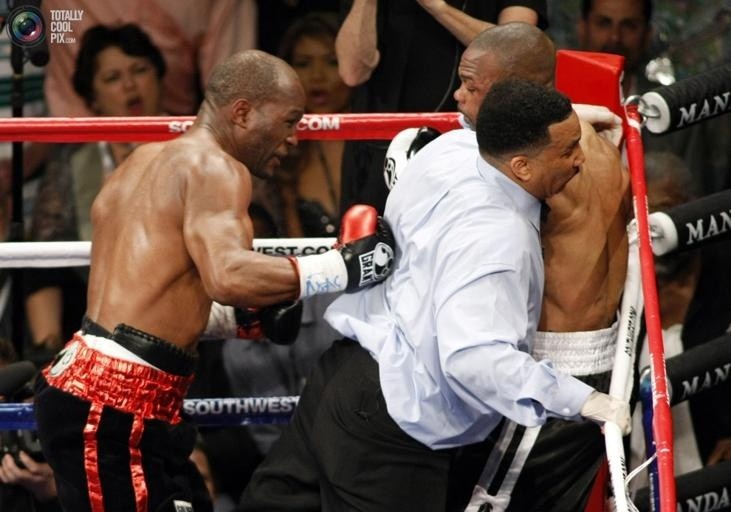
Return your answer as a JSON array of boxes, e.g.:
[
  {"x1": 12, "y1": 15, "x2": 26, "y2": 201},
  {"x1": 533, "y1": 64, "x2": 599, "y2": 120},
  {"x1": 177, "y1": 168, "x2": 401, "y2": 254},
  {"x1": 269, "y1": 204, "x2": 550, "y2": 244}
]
[{"x1": 1, "y1": 429, "x2": 46, "y2": 468}]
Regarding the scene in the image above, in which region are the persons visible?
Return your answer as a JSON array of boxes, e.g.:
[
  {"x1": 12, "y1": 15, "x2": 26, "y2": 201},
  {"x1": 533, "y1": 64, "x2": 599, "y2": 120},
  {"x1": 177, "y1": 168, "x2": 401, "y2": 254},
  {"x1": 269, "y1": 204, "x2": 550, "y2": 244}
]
[{"x1": 0, "y1": 0, "x2": 731, "y2": 512}]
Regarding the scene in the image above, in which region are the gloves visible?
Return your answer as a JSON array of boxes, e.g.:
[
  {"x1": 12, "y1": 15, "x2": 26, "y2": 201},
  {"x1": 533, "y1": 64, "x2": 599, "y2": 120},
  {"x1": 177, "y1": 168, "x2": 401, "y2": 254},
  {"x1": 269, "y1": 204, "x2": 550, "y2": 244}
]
[
  {"x1": 198, "y1": 298, "x2": 303, "y2": 345},
  {"x1": 284, "y1": 204, "x2": 396, "y2": 302},
  {"x1": 579, "y1": 390, "x2": 632, "y2": 437},
  {"x1": 383, "y1": 125, "x2": 441, "y2": 191}
]
